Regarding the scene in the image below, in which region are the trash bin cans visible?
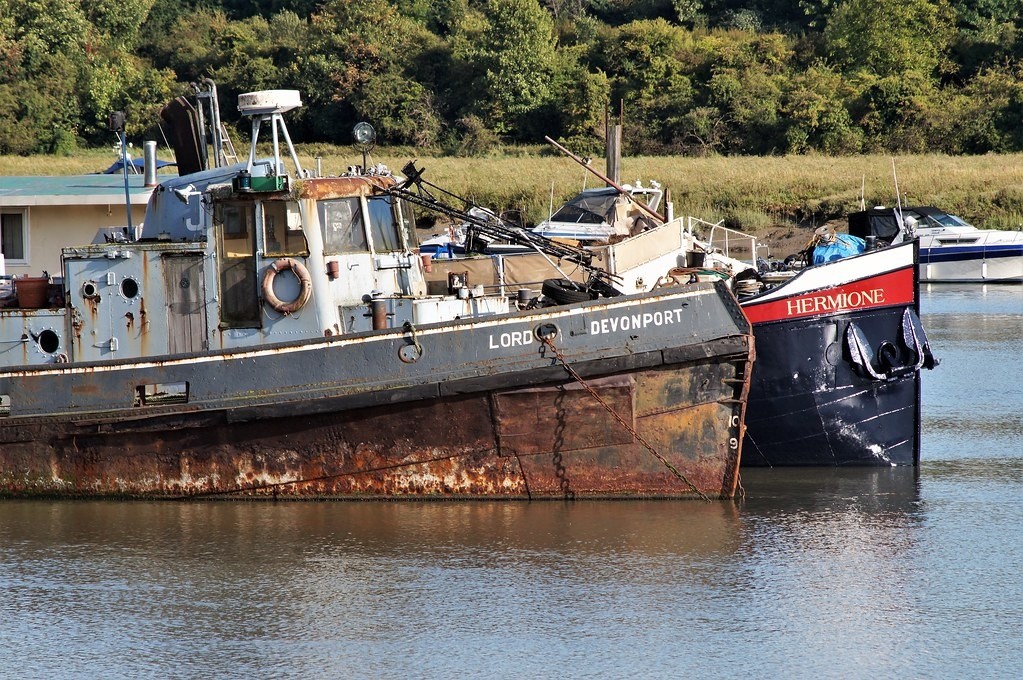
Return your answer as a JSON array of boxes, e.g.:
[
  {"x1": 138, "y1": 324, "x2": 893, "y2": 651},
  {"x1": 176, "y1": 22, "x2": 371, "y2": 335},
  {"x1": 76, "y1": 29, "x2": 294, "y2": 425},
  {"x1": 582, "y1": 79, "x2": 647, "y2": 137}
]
[
  {"x1": 686, "y1": 251, "x2": 706, "y2": 268},
  {"x1": 13, "y1": 274, "x2": 50, "y2": 309}
]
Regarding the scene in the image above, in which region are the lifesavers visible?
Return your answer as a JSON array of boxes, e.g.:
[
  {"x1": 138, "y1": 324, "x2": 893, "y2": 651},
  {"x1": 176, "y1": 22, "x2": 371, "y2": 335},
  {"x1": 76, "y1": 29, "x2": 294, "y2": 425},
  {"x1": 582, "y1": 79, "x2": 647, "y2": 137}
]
[{"x1": 263, "y1": 258, "x2": 313, "y2": 312}]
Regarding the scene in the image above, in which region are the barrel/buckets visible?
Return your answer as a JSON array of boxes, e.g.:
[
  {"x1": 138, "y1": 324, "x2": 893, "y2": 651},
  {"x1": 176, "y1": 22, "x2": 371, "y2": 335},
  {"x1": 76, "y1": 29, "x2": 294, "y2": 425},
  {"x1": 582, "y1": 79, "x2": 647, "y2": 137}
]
[{"x1": 15, "y1": 278, "x2": 48, "y2": 309}]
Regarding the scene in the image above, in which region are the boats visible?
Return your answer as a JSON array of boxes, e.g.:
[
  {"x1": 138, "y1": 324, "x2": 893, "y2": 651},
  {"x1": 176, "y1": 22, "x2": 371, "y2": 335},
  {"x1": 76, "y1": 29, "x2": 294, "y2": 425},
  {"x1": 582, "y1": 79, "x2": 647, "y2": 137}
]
[
  {"x1": 419, "y1": 95, "x2": 770, "y2": 293},
  {"x1": 460, "y1": 157, "x2": 940, "y2": 466},
  {"x1": 850, "y1": 158, "x2": 1023, "y2": 286},
  {"x1": 0, "y1": 66, "x2": 756, "y2": 503}
]
